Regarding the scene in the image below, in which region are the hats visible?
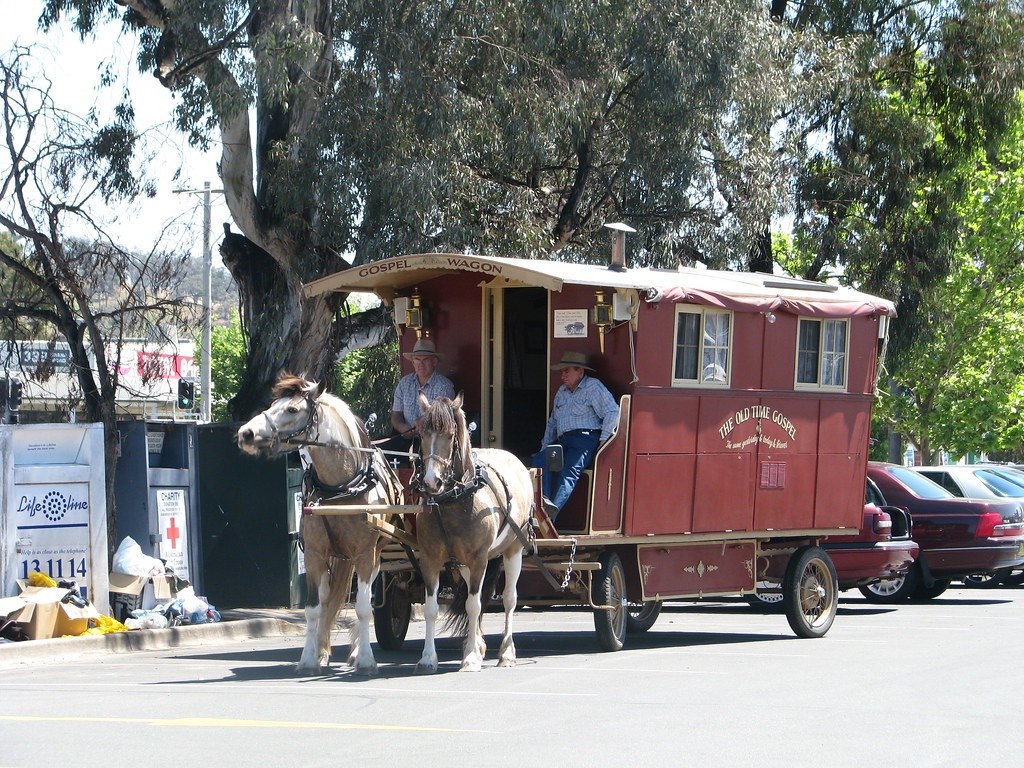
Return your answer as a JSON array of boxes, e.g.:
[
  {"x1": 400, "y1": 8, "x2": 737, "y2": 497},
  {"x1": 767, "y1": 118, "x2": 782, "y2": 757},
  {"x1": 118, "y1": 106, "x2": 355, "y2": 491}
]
[
  {"x1": 703, "y1": 363, "x2": 726, "y2": 382},
  {"x1": 551, "y1": 352, "x2": 597, "y2": 372},
  {"x1": 403, "y1": 340, "x2": 448, "y2": 368}
]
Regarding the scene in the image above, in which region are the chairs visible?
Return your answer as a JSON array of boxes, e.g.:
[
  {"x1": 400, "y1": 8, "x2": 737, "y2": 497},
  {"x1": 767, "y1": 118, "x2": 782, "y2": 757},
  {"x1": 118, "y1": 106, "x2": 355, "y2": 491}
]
[{"x1": 552, "y1": 392, "x2": 631, "y2": 534}]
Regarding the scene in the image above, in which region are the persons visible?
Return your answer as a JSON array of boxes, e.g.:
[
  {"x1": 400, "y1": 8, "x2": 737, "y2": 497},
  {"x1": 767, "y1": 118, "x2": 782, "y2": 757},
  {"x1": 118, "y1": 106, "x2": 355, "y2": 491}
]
[
  {"x1": 378, "y1": 339, "x2": 455, "y2": 468},
  {"x1": 531, "y1": 352, "x2": 620, "y2": 524}
]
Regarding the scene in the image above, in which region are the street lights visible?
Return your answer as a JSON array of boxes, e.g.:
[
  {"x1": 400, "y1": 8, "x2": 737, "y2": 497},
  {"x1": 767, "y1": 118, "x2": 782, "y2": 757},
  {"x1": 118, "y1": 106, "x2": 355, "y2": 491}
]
[{"x1": 179, "y1": 181, "x2": 227, "y2": 422}]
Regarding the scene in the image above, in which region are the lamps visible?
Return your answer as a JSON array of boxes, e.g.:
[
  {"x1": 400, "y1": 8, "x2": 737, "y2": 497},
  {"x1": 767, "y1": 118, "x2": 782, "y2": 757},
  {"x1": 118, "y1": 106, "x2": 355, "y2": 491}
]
[
  {"x1": 591, "y1": 290, "x2": 613, "y2": 352},
  {"x1": 405, "y1": 287, "x2": 427, "y2": 341}
]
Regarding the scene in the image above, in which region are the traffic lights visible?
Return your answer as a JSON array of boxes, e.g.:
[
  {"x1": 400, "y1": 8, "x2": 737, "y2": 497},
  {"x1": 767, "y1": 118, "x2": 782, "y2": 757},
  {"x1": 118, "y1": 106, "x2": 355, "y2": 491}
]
[{"x1": 178, "y1": 379, "x2": 195, "y2": 410}]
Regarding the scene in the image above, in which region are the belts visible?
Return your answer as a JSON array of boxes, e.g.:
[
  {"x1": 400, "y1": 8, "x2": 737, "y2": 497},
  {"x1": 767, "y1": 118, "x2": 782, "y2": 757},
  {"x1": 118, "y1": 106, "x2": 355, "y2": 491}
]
[{"x1": 564, "y1": 429, "x2": 601, "y2": 435}]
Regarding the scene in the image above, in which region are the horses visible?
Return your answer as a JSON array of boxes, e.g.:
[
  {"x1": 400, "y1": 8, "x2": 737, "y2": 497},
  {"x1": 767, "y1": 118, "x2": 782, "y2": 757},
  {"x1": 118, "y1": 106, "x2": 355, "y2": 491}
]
[
  {"x1": 234, "y1": 368, "x2": 405, "y2": 676},
  {"x1": 413, "y1": 388, "x2": 535, "y2": 671}
]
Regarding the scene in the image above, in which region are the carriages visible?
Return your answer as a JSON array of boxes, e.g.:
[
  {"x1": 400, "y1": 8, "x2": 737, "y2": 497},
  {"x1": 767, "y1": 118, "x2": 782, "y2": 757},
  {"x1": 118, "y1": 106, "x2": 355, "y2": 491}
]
[{"x1": 237, "y1": 222, "x2": 898, "y2": 677}]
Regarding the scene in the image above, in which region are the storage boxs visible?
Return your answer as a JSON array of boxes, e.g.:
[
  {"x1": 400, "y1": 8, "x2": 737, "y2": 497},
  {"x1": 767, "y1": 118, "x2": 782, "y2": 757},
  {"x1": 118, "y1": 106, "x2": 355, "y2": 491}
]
[
  {"x1": 0, "y1": 579, "x2": 99, "y2": 639},
  {"x1": 108, "y1": 569, "x2": 173, "y2": 624}
]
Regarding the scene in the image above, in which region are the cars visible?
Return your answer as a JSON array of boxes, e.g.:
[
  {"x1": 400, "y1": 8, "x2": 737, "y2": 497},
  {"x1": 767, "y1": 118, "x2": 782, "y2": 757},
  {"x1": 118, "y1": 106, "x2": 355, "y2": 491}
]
[
  {"x1": 740, "y1": 475, "x2": 920, "y2": 614},
  {"x1": 867, "y1": 461, "x2": 1024, "y2": 600},
  {"x1": 911, "y1": 463, "x2": 1024, "y2": 499}
]
